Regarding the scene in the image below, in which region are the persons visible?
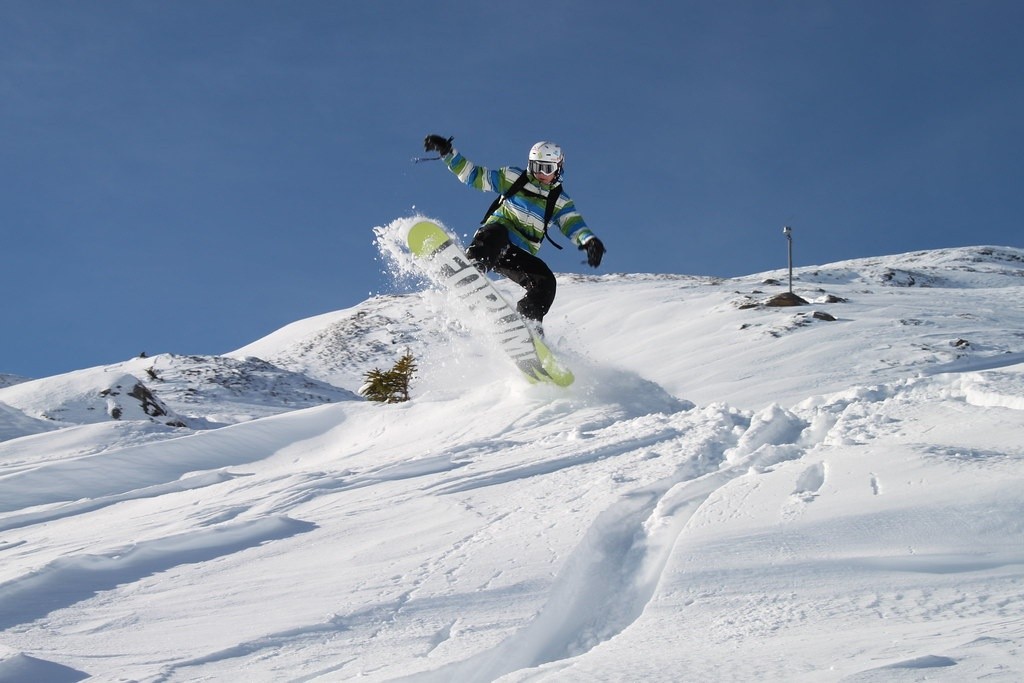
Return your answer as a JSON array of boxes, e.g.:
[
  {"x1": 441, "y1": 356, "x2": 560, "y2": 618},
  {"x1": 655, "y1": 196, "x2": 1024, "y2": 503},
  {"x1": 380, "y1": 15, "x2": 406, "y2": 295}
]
[{"x1": 423, "y1": 134, "x2": 606, "y2": 338}]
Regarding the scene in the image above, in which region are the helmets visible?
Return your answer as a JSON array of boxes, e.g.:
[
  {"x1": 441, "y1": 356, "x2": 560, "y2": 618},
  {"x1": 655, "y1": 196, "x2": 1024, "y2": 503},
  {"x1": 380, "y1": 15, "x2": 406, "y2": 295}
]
[{"x1": 529, "y1": 141, "x2": 565, "y2": 164}]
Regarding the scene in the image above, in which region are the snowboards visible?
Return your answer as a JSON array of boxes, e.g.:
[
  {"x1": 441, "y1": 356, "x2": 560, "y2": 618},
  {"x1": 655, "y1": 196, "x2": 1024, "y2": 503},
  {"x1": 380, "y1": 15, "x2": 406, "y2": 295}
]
[{"x1": 408, "y1": 220, "x2": 573, "y2": 392}]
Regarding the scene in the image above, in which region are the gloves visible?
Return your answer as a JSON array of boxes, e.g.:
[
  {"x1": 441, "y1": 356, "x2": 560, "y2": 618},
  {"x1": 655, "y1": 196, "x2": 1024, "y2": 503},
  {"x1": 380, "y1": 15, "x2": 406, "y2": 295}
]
[
  {"x1": 424, "y1": 134, "x2": 454, "y2": 155},
  {"x1": 578, "y1": 238, "x2": 606, "y2": 268}
]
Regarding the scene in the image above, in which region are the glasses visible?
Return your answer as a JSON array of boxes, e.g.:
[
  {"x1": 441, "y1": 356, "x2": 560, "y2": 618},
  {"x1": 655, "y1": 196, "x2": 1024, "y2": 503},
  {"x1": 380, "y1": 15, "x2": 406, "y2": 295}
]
[{"x1": 530, "y1": 160, "x2": 558, "y2": 176}]
